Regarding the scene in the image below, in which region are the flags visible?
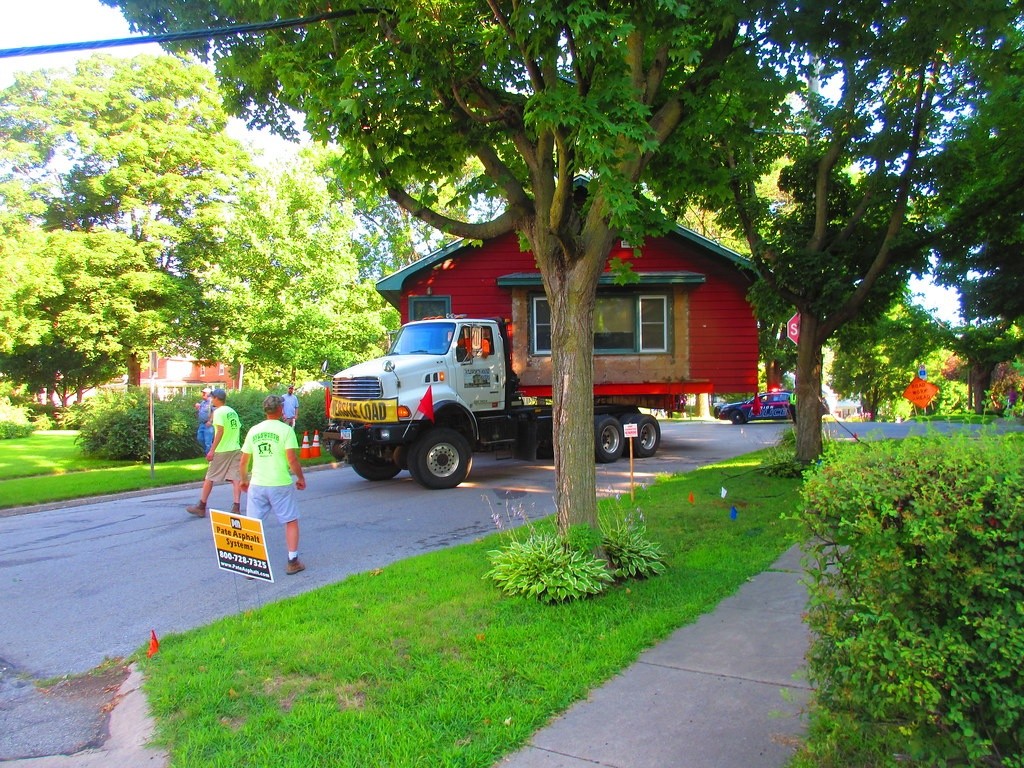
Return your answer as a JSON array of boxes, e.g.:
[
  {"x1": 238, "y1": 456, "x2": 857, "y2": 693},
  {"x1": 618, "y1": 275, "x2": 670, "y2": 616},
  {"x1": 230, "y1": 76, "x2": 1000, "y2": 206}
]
[
  {"x1": 418, "y1": 387, "x2": 435, "y2": 423},
  {"x1": 325, "y1": 388, "x2": 330, "y2": 419}
]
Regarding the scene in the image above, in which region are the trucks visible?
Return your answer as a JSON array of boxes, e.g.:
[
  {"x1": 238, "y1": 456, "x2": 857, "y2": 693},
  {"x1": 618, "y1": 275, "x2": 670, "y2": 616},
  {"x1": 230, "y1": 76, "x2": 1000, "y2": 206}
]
[{"x1": 325, "y1": 315, "x2": 715, "y2": 489}]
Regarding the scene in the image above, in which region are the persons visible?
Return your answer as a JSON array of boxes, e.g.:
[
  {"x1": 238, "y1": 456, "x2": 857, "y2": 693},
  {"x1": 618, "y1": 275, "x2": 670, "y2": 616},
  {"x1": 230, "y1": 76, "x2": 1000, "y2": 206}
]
[
  {"x1": 187, "y1": 388, "x2": 241, "y2": 518},
  {"x1": 281, "y1": 386, "x2": 298, "y2": 427},
  {"x1": 457, "y1": 327, "x2": 489, "y2": 360},
  {"x1": 788, "y1": 389, "x2": 796, "y2": 425},
  {"x1": 238, "y1": 395, "x2": 306, "y2": 575}
]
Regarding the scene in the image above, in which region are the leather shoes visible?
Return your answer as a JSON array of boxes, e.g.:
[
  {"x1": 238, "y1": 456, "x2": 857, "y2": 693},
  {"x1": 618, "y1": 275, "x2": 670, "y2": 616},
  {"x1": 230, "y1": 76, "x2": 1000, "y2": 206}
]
[
  {"x1": 230, "y1": 508, "x2": 239, "y2": 514},
  {"x1": 187, "y1": 505, "x2": 204, "y2": 517}
]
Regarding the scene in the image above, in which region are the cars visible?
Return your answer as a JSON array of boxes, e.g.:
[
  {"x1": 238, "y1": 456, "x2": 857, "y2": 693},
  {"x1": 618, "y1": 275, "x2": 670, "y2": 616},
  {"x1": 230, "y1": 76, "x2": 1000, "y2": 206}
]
[{"x1": 719, "y1": 389, "x2": 791, "y2": 424}]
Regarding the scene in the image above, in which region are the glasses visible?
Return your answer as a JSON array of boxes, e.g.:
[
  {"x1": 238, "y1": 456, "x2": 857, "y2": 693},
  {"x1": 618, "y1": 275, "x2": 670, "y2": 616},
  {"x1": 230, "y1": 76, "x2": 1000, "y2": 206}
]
[{"x1": 289, "y1": 388, "x2": 293, "y2": 389}]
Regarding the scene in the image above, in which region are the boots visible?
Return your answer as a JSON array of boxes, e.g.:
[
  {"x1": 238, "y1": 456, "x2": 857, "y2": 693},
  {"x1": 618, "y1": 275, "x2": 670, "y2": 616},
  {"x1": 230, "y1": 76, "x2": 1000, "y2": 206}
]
[{"x1": 286, "y1": 555, "x2": 305, "y2": 575}]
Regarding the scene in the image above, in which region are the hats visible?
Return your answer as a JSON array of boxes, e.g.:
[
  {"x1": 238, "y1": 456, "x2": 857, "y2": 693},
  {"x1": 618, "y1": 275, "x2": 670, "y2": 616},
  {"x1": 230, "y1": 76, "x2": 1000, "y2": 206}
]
[
  {"x1": 210, "y1": 389, "x2": 226, "y2": 400},
  {"x1": 263, "y1": 395, "x2": 285, "y2": 412},
  {"x1": 202, "y1": 387, "x2": 211, "y2": 392}
]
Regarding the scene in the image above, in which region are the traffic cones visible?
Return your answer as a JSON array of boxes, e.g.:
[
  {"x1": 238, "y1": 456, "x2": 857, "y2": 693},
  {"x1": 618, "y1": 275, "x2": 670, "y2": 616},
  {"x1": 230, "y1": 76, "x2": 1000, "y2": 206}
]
[
  {"x1": 310, "y1": 430, "x2": 321, "y2": 458},
  {"x1": 300, "y1": 430, "x2": 312, "y2": 460}
]
